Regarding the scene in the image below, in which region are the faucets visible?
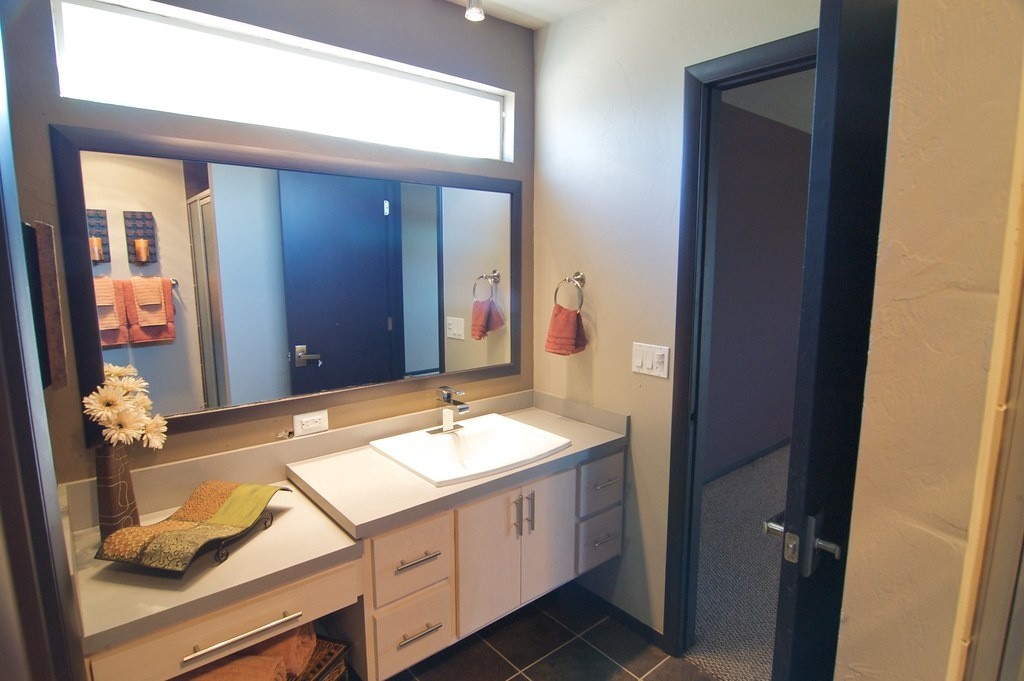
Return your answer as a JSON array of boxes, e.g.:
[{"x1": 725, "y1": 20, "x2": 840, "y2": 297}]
[{"x1": 433, "y1": 384, "x2": 471, "y2": 433}]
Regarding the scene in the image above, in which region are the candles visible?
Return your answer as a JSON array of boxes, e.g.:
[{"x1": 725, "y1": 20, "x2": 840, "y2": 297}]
[
  {"x1": 134, "y1": 237, "x2": 150, "y2": 265},
  {"x1": 88, "y1": 233, "x2": 105, "y2": 264}
]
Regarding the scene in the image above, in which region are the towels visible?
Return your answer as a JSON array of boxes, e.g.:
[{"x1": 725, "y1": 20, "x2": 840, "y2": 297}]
[
  {"x1": 94, "y1": 275, "x2": 177, "y2": 352},
  {"x1": 172, "y1": 655, "x2": 288, "y2": 681},
  {"x1": 239, "y1": 621, "x2": 319, "y2": 677},
  {"x1": 544, "y1": 304, "x2": 590, "y2": 358},
  {"x1": 469, "y1": 298, "x2": 507, "y2": 342}
]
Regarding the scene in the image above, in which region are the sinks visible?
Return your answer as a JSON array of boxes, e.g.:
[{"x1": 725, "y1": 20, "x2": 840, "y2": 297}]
[{"x1": 367, "y1": 410, "x2": 572, "y2": 490}]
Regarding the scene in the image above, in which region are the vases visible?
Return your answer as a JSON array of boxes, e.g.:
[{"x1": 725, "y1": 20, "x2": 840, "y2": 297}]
[{"x1": 92, "y1": 442, "x2": 141, "y2": 564}]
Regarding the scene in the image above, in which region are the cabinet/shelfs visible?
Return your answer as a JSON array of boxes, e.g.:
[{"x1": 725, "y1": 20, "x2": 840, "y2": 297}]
[
  {"x1": 455, "y1": 466, "x2": 576, "y2": 642},
  {"x1": 61, "y1": 478, "x2": 365, "y2": 680},
  {"x1": 576, "y1": 448, "x2": 622, "y2": 579},
  {"x1": 365, "y1": 511, "x2": 454, "y2": 681}
]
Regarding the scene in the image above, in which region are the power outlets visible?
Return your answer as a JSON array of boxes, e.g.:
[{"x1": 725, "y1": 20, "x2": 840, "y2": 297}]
[{"x1": 291, "y1": 409, "x2": 329, "y2": 438}]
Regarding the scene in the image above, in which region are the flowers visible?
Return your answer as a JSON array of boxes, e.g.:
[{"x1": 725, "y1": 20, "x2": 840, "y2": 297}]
[
  {"x1": 83, "y1": 385, "x2": 168, "y2": 452},
  {"x1": 99, "y1": 361, "x2": 150, "y2": 400}
]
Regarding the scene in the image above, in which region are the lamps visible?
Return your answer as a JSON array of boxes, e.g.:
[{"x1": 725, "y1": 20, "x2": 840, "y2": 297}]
[{"x1": 464, "y1": 0, "x2": 486, "y2": 24}]
